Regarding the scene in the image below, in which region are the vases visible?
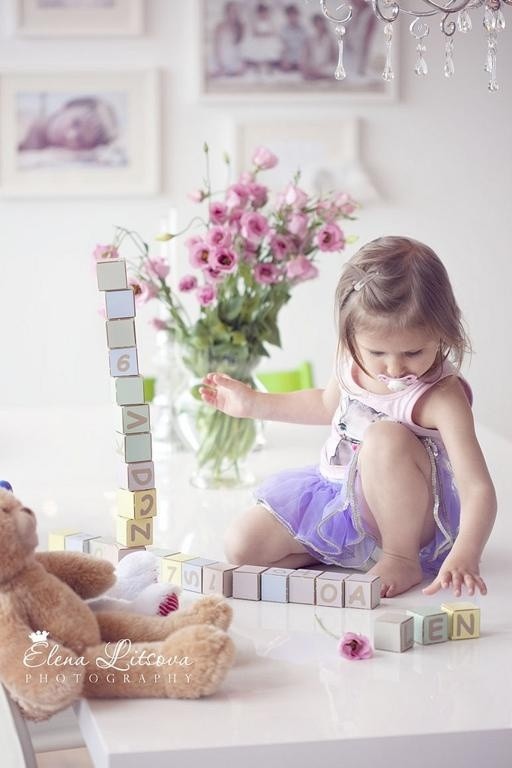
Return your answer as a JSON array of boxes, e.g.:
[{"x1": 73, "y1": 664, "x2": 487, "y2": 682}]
[
  {"x1": 91, "y1": 139, "x2": 362, "y2": 473},
  {"x1": 170, "y1": 344, "x2": 274, "y2": 500}
]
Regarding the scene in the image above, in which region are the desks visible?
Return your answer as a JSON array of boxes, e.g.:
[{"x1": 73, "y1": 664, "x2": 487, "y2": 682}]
[{"x1": 70, "y1": 560, "x2": 510, "y2": 768}]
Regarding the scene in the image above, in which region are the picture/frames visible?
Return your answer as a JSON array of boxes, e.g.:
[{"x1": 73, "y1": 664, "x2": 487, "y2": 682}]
[
  {"x1": 0, "y1": 0, "x2": 401, "y2": 194},
  {"x1": 70, "y1": 560, "x2": 510, "y2": 768}
]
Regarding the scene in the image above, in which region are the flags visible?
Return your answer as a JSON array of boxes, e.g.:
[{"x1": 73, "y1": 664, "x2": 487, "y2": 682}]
[
  {"x1": 170, "y1": 344, "x2": 274, "y2": 500},
  {"x1": 91, "y1": 139, "x2": 362, "y2": 473}
]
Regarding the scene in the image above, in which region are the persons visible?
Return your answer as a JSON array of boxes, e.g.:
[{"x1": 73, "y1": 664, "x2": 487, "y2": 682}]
[
  {"x1": 197, "y1": 235, "x2": 499, "y2": 599},
  {"x1": 20, "y1": 96, "x2": 118, "y2": 153},
  {"x1": 210, "y1": 0, "x2": 379, "y2": 85}
]
